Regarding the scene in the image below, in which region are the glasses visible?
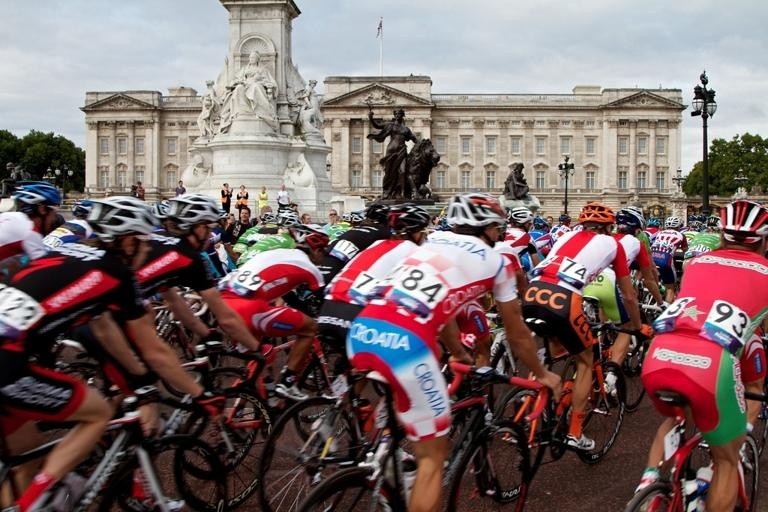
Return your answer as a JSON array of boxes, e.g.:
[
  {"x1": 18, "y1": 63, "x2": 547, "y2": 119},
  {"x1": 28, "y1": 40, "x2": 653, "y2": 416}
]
[{"x1": 330, "y1": 214, "x2": 336, "y2": 216}]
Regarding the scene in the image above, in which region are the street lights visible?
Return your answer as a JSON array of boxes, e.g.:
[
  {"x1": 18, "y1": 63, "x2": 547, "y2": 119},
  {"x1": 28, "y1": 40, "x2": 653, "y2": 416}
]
[
  {"x1": 733, "y1": 166, "x2": 747, "y2": 189},
  {"x1": 689, "y1": 69, "x2": 720, "y2": 216},
  {"x1": 671, "y1": 168, "x2": 685, "y2": 193},
  {"x1": 54, "y1": 163, "x2": 73, "y2": 198},
  {"x1": 557, "y1": 156, "x2": 574, "y2": 219}
]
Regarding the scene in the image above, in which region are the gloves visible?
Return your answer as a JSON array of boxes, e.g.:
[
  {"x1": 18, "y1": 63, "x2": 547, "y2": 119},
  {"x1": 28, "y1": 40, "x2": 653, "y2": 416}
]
[
  {"x1": 258, "y1": 343, "x2": 278, "y2": 367},
  {"x1": 203, "y1": 328, "x2": 226, "y2": 348},
  {"x1": 195, "y1": 390, "x2": 226, "y2": 418}
]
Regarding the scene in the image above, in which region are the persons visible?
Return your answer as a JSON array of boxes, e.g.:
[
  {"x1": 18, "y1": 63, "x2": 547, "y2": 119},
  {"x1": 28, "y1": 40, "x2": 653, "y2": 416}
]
[
  {"x1": 650, "y1": 217, "x2": 688, "y2": 311},
  {"x1": 250, "y1": 206, "x2": 272, "y2": 227},
  {"x1": 427, "y1": 206, "x2": 455, "y2": 235},
  {"x1": 456, "y1": 193, "x2": 530, "y2": 368},
  {"x1": 0, "y1": 181, "x2": 63, "y2": 262},
  {"x1": 217, "y1": 231, "x2": 331, "y2": 402},
  {"x1": 645, "y1": 218, "x2": 663, "y2": 235},
  {"x1": 0, "y1": 194, "x2": 227, "y2": 512},
  {"x1": 625, "y1": 198, "x2": 768, "y2": 512},
  {"x1": 258, "y1": 186, "x2": 268, "y2": 214},
  {"x1": 323, "y1": 209, "x2": 338, "y2": 227},
  {"x1": 233, "y1": 212, "x2": 275, "y2": 257},
  {"x1": 220, "y1": 51, "x2": 278, "y2": 134},
  {"x1": 504, "y1": 164, "x2": 529, "y2": 200},
  {"x1": 247, "y1": 207, "x2": 301, "y2": 247},
  {"x1": 571, "y1": 223, "x2": 584, "y2": 231},
  {"x1": 42, "y1": 199, "x2": 98, "y2": 251},
  {"x1": 291, "y1": 204, "x2": 298, "y2": 209},
  {"x1": 519, "y1": 216, "x2": 553, "y2": 273},
  {"x1": 299, "y1": 79, "x2": 324, "y2": 134},
  {"x1": 276, "y1": 184, "x2": 291, "y2": 207},
  {"x1": 301, "y1": 214, "x2": 311, "y2": 224},
  {"x1": 545, "y1": 216, "x2": 553, "y2": 225},
  {"x1": 221, "y1": 183, "x2": 233, "y2": 214},
  {"x1": 682, "y1": 220, "x2": 705, "y2": 249},
  {"x1": 63, "y1": 191, "x2": 277, "y2": 512},
  {"x1": 149, "y1": 204, "x2": 171, "y2": 224},
  {"x1": 504, "y1": 206, "x2": 540, "y2": 281},
  {"x1": 327, "y1": 215, "x2": 364, "y2": 246},
  {"x1": 175, "y1": 180, "x2": 186, "y2": 196},
  {"x1": 369, "y1": 109, "x2": 418, "y2": 200},
  {"x1": 431, "y1": 215, "x2": 440, "y2": 226},
  {"x1": 1, "y1": 162, "x2": 23, "y2": 196},
  {"x1": 682, "y1": 217, "x2": 722, "y2": 271},
  {"x1": 19, "y1": 169, "x2": 31, "y2": 181},
  {"x1": 235, "y1": 185, "x2": 249, "y2": 220},
  {"x1": 582, "y1": 205, "x2": 671, "y2": 409},
  {"x1": 346, "y1": 192, "x2": 562, "y2": 512},
  {"x1": 321, "y1": 203, "x2": 397, "y2": 286},
  {"x1": 198, "y1": 209, "x2": 237, "y2": 287},
  {"x1": 549, "y1": 214, "x2": 572, "y2": 243},
  {"x1": 522, "y1": 201, "x2": 655, "y2": 451},
  {"x1": 326, "y1": 212, "x2": 352, "y2": 238},
  {"x1": 612, "y1": 224, "x2": 663, "y2": 289},
  {"x1": 131, "y1": 181, "x2": 144, "y2": 201},
  {"x1": 738, "y1": 317, "x2": 768, "y2": 475},
  {"x1": 197, "y1": 80, "x2": 217, "y2": 137},
  {"x1": 226, "y1": 206, "x2": 252, "y2": 244},
  {"x1": 317, "y1": 202, "x2": 431, "y2": 475},
  {"x1": 236, "y1": 224, "x2": 324, "y2": 307}
]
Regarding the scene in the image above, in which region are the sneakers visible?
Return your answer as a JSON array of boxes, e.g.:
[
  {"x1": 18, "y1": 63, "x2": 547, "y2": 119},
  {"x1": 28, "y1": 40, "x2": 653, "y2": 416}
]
[
  {"x1": 564, "y1": 433, "x2": 596, "y2": 451},
  {"x1": 634, "y1": 471, "x2": 660, "y2": 493},
  {"x1": 602, "y1": 372, "x2": 619, "y2": 407},
  {"x1": 267, "y1": 389, "x2": 280, "y2": 407},
  {"x1": 738, "y1": 440, "x2": 753, "y2": 471},
  {"x1": 15, "y1": 472, "x2": 89, "y2": 511},
  {"x1": 276, "y1": 377, "x2": 310, "y2": 401},
  {"x1": 126, "y1": 494, "x2": 185, "y2": 512}
]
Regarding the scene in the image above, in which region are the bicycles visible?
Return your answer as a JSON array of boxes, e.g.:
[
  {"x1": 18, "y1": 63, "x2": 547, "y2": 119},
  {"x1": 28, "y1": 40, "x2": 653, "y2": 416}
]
[
  {"x1": 115, "y1": 340, "x2": 274, "y2": 511},
  {"x1": 482, "y1": 317, "x2": 627, "y2": 504},
  {"x1": 260, "y1": 351, "x2": 456, "y2": 511},
  {"x1": 294, "y1": 368, "x2": 548, "y2": 512},
  {"x1": 0, "y1": 384, "x2": 230, "y2": 511},
  {"x1": 624, "y1": 388, "x2": 768, "y2": 511},
  {"x1": 580, "y1": 265, "x2": 676, "y2": 411},
  {"x1": 52, "y1": 276, "x2": 234, "y2": 382},
  {"x1": 179, "y1": 299, "x2": 350, "y2": 481}
]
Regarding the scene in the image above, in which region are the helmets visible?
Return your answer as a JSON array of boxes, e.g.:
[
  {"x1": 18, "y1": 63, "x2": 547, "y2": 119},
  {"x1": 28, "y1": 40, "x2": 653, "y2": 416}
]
[
  {"x1": 259, "y1": 212, "x2": 275, "y2": 223},
  {"x1": 688, "y1": 215, "x2": 696, "y2": 222},
  {"x1": 559, "y1": 214, "x2": 571, "y2": 221},
  {"x1": 533, "y1": 216, "x2": 549, "y2": 227},
  {"x1": 446, "y1": 192, "x2": 506, "y2": 228},
  {"x1": 162, "y1": 191, "x2": 228, "y2": 226},
  {"x1": 305, "y1": 231, "x2": 330, "y2": 249},
  {"x1": 578, "y1": 202, "x2": 615, "y2": 225},
  {"x1": 78, "y1": 195, "x2": 159, "y2": 240},
  {"x1": 646, "y1": 218, "x2": 661, "y2": 228},
  {"x1": 720, "y1": 199, "x2": 768, "y2": 245},
  {"x1": 14, "y1": 182, "x2": 62, "y2": 207},
  {"x1": 384, "y1": 202, "x2": 431, "y2": 235},
  {"x1": 696, "y1": 215, "x2": 706, "y2": 224},
  {"x1": 506, "y1": 207, "x2": 535, "y2": 224},
  {"x1": 276, "y1": 207, "x2": 300, "y2": 227},
  {"x1": 706, "y1": 216, "x2": 721, "y2": 229},
  {"x1": 664, "y1": 217, "x2": 681, "y2": 229},
  {"x1": 616, "y1": 207, "x2": 645, "y2": 235}
]
